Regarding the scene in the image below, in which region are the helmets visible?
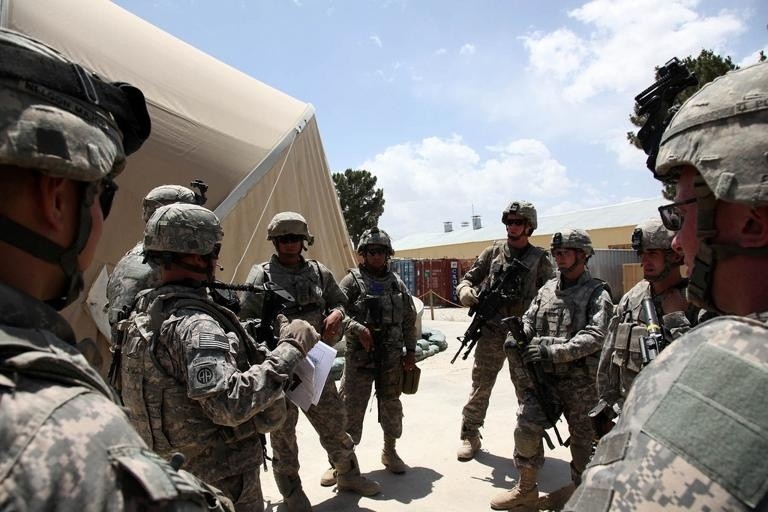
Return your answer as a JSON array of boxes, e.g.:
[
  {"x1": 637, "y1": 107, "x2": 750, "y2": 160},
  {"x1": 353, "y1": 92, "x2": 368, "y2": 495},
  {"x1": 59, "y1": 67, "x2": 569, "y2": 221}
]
[
  {"x1": 142, "y1": 201, "x2": 224, "y2": 263},
  {"x1": 266, "y1": 211, "x2": 314, "y2": 245},
  {"x1": 502, "y1": 200, "x2": 537, "y2": 229},
  {"x1": 357, "y1": 226, "x2": 392, "y2": 253},
  {"x1": 0, "y1": 27, "x2": 151, "y2": 181},
  {"x1": 655, "y1": 60, "x2": 768, "y2": 207},
  {"x1": 631, "y1": 222, "x2": 675, "y2": 250},
  {"x1": 550, "y1": 228, "x2": 594, "y2": 257},
  {"x1": 143, "y1": 179, "x2": 208, "y2": 222}
]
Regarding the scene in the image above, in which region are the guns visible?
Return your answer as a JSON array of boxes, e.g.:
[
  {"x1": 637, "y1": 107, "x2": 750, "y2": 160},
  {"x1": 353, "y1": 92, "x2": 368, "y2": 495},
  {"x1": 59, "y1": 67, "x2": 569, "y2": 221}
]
[
  {"x1": 362, "y1": 298, "x2": 383, "y2": 423},
  {"x1": 257, "y1": 280, "x2": 294, "y2": 470},
  {"x1": 588, "y1": 401, "x2": 613, "y2": 460},
  {"x1": 638, "y1": 296, "x2": 670, "y2": 367},
  {"x1": 450, "y1": 258, "x2": 529, "y2": 366},
  {"x1": 500, "y1": 317, "x2": 563, "y2": 448}
]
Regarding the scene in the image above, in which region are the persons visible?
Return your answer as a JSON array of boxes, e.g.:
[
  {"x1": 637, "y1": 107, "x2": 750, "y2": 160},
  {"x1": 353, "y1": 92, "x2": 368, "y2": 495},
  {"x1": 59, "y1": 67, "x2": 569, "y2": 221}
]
[
  {"x1": 107, "y1": 181, "x2": 416, "y2": 511},
  {"x1": 457, "y1": 202, "x2": 700, "y2": 512},
  {"x1": 564, "y1": 59, "x2": 767, "y2": 512},
  {"x1": 1, "y1": 28, "x2": 239, "y2": 512}
]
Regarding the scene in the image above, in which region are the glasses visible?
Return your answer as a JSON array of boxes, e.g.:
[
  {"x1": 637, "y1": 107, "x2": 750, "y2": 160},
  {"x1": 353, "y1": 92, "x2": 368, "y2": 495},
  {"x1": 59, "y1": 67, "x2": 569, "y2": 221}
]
[
  {"x1": 503, "y1": 219, "x2": 526, "y2": 225},
  {"x1": 278, "y1": 235, "x2": 303, "y2": 243},
  {"x1": 365, "y1": 247, "x2": 387, "y2": 256},
  {"x1": 658, "y1": 198, "x2": 696, "y2": 230},
  {"x1": 100, "y1": 178, "x2": 118, "y2": 220}
]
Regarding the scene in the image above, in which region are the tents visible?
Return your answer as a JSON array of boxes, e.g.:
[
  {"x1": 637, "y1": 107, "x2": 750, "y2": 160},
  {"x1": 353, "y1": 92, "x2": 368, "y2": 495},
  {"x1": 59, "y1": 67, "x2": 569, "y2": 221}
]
[{"x1": 1, "y1": 0, "x2": 359, "y2": 287}]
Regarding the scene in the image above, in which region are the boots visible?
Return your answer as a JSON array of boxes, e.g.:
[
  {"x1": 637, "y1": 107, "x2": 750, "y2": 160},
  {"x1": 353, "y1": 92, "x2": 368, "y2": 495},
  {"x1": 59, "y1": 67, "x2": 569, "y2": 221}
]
[
  {"x1": 382, "y1": 436, "x2": 406, "y2": 472},
  {"x1": 491, "y1": 469, "x2": 538, "y2": 509},
  {"x1": 458, "y1": 422, "x2": 481, "y2": 460},
  {"x1": 321, "y1": 468, "x2": 337, "y2": 485},
  {"x1": 335, "y1": 453, "x2": 380, "y2": 495},
  {"x1": 276, "y1": 473, "x2": 311, "y2": 512}
]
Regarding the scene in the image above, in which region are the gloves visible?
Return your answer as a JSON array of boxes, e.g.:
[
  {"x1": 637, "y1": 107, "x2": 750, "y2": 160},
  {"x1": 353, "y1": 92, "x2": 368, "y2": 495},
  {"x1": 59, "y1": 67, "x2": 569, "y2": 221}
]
[
  {"x1": 459, "y1": 285, "x2": 478, "y2": 307},
  {"x1": 521, "y1": 345, "x2": 550, "y2": 364},
  {"x1": 277, "y1": 314, "x2": 321, "y2": 356}
]
